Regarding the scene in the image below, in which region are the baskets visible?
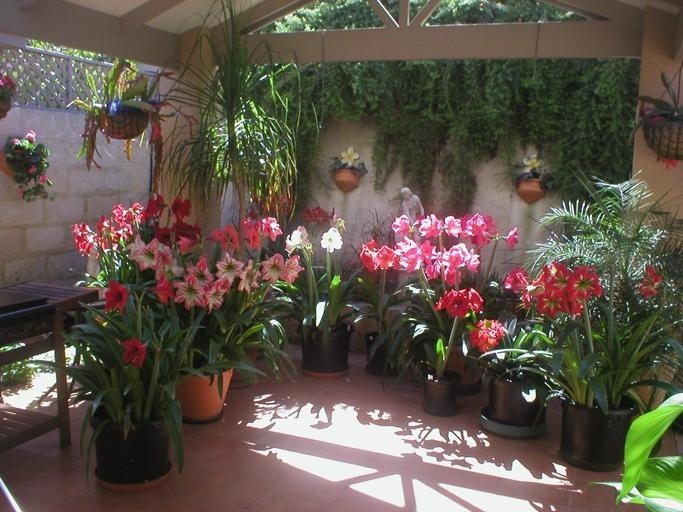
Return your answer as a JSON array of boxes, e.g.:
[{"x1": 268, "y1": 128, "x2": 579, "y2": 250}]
[
  {"x1": 644, "y1": 116, "x2": 682, "y2": 161},
  {"x1": 96, "y1": 106, "x2": 149, "y2": 139}
]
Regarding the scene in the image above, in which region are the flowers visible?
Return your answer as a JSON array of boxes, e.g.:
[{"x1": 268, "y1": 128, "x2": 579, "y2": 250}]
[{"x1": 0, "y1": 57, "x2": 683, "y2": 493}]
